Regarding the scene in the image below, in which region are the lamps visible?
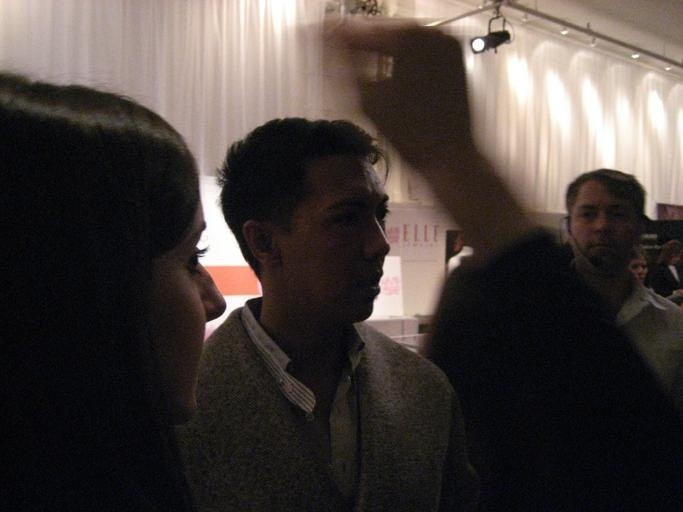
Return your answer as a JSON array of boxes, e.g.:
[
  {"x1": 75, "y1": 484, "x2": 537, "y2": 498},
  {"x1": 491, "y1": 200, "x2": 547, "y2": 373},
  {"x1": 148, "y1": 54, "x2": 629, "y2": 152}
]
[{"x1": 470, "y1": 7, "x2": 516, "y2": 54}]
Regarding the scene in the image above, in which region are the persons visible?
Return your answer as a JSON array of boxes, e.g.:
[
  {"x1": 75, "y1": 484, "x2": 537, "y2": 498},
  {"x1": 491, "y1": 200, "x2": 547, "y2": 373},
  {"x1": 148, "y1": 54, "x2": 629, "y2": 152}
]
[
  {"x1": 560, "y1": 167, "x2": 683, "y2": 414},
  {"x1": 627, "y1": 245, "x2": 649, "y2": 285},
  {"x1": 299, "y1": 13, "x2": 683, "y2": 511},
  {"x1": 644, "y1": 238, "x2": 683, "y2": 308},
  {"x1": 0, "y1": 64, "x2": 227, "y2": 512},
  {"x1": 177, "y1": 114, "x2": 483, "y2": 512}
]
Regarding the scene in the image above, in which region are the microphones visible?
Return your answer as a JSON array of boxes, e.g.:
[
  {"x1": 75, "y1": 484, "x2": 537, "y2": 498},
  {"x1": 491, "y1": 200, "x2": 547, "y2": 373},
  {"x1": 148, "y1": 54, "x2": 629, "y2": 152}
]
[{"x1": 566, "y1": 217, "x2": 603, "y2": 267}]
